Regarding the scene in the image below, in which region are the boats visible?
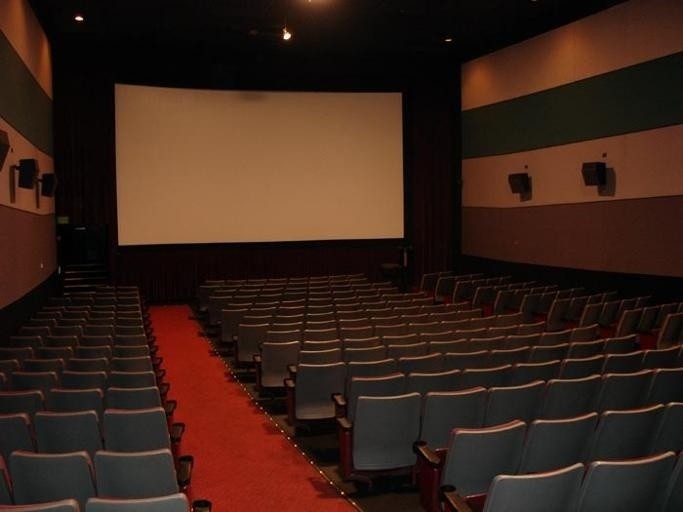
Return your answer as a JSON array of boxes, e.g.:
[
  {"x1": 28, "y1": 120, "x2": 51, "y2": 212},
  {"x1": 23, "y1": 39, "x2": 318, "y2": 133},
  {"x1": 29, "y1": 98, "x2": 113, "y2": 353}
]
[
  {"x1": 1, "y1": 129, "x2": 12, "y2": 168},
  {"x1": 507, "y1": 173, "x2": 530, "y2": 195},
  {"x1": 41, "y1": 172, "x2": 60, "y2": 198},
  {"x1": 16, "y1": 158, "x2": 40, "y2": 189},
  {"x1": 581, "y1": 162, "x2": 606, "y2": 187}
]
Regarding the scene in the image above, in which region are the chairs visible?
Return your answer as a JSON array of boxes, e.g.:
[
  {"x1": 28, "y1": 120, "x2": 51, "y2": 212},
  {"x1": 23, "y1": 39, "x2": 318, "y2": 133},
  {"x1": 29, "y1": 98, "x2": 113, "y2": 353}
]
[
  {"x1": 0, "y1": 283, "x2": 212, "y2": 512},
  {"x1": 187, "y1": 270, "x2": 683, "y2": 512}
]
